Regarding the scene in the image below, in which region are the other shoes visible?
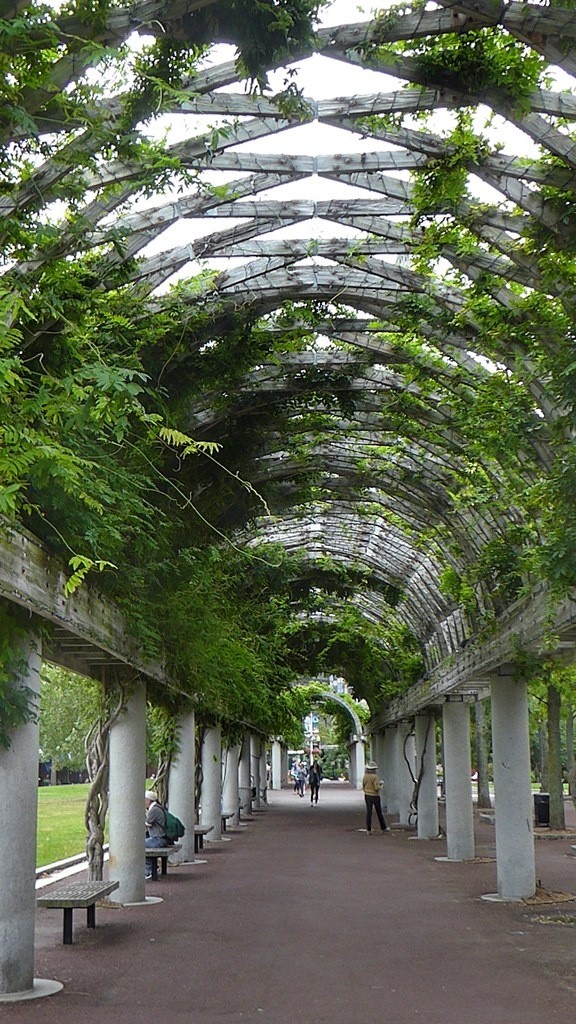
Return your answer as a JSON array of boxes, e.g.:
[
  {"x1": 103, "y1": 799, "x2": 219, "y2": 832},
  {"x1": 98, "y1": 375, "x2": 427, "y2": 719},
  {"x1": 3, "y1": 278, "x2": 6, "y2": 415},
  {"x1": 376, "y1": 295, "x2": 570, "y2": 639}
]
[
  {"x1": 299, "y1": 794, "x2": 303, "y2": 797},
  {"x1": 366, "y1": 831, "x2": 374, "y2": 835},
  {"x1": 311, "y1": 801, "x2": 313, "y2": 806},
  {"x1": 145, "y1": 875, "x2": 152, "y2": 879},
  {"x1": 383, "y1": 827, "x2": 390, "y2": 833},
  {"x1": 315, "y1": 802, "x2": 318, "y2": 806}
]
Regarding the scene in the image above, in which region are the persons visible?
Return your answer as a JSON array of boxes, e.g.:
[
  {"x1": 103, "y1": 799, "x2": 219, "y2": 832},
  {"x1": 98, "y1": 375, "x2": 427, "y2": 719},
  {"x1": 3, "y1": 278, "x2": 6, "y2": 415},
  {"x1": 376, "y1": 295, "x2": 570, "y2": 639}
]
[
  {"x1": 290, "y1": 761, "x2": 308, "y2": 797},
  {"x1": 363, "y1": 761, "x2": 391, "y2": 835},
  {"x1": 144, "y1": 790, "x2": 167, "y2": 879},
  {"x1": 309, "y1": 760, "x2": 323, "y2": 807}
]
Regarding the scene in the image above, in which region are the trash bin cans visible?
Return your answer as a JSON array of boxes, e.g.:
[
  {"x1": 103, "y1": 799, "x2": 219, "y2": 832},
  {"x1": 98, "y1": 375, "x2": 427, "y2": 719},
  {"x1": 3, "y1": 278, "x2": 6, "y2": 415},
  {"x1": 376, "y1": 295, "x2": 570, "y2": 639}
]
[{"x1": 533, "y1": 792, "x2": 550, "y2": 827}]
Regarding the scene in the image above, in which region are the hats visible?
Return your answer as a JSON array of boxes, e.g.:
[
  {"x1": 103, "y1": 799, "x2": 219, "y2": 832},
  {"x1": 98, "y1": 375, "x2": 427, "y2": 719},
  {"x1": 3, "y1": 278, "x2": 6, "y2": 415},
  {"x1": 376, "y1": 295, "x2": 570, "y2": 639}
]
[
  {"x1": 367, "y1": 762, "x2": 378, "y2": 769},
  {"x1": 145, "y1": 791, "x2": 158, "y2": 800}
]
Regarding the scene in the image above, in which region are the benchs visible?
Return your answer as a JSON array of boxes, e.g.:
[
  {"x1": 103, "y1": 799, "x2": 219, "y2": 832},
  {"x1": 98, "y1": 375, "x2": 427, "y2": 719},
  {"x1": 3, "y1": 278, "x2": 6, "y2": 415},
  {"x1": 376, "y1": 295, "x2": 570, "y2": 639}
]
[
  {"x1": 36, "y1": 881, "x2": 120, "y2": 945},
  {"x1": 221, "y1": 812, "x2": 235, "y2": 834},
  {"x1": 239, "y1": 803, "x2": 247, "y2": 820},
  {"x1": 250, "y1": 797, "x2": 258, "y2": 811},
  {"x1": 145, "y1": 844, "x2": 183, "y2": 881},
  {"x1": 194, "y1": 825, "x2": 214, "y2": 854}
]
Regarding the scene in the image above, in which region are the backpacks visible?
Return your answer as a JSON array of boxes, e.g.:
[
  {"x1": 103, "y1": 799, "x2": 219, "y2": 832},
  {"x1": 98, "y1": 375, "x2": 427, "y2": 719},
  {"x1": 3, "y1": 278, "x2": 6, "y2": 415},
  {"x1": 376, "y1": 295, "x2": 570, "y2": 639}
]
[{"x1": 154, "y1": 805, "x2": 185, "y2": 841}]
